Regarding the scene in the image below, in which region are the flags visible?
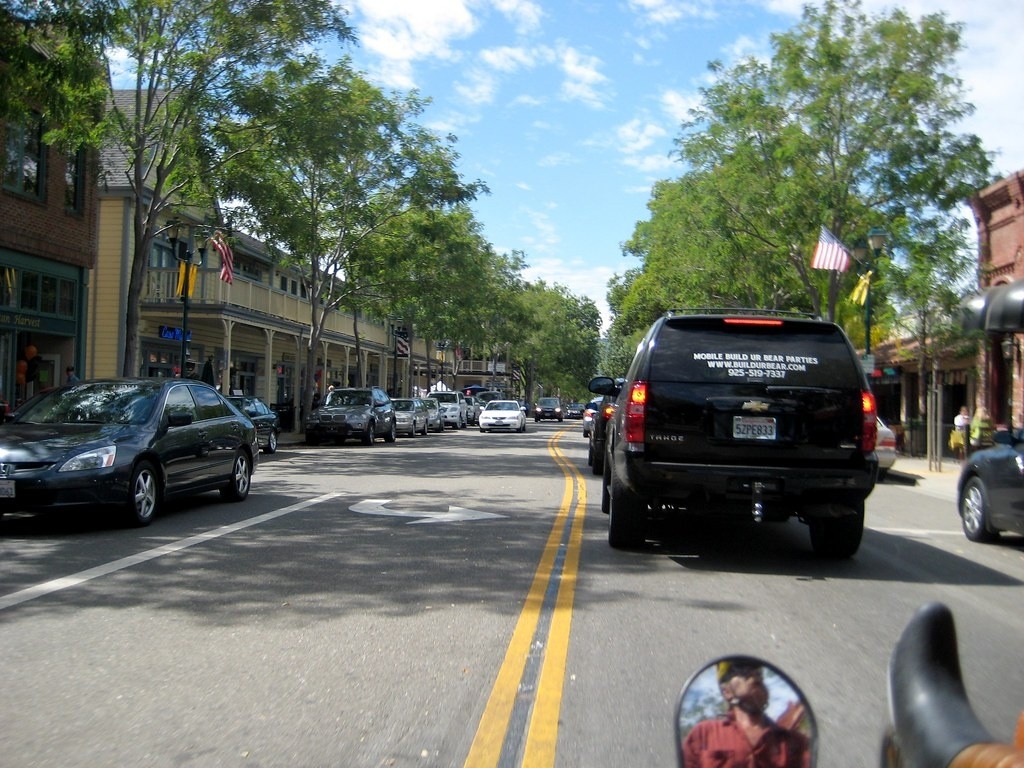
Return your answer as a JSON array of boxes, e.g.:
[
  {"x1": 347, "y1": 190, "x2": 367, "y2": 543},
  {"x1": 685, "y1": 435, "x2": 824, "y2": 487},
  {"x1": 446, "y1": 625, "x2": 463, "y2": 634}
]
[{"x1": 812, "y1": 225, "x2": 847, "y2": 272}]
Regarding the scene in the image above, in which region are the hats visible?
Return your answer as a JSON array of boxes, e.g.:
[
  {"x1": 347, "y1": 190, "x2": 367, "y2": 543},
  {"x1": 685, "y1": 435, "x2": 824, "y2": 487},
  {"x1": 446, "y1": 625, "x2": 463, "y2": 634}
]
[{"x1": 717, "y1": 659, "x2": 764, "y2": 684}]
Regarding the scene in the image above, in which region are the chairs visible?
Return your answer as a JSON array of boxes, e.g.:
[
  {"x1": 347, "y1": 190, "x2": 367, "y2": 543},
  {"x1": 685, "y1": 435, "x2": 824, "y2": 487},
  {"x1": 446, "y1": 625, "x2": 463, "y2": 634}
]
[
  {"x1": 970, "y1": 426, "x2": 998, "y2": 448},
  {"x1": 132, "y1": 398, "x2": 155, "y2": 420}
]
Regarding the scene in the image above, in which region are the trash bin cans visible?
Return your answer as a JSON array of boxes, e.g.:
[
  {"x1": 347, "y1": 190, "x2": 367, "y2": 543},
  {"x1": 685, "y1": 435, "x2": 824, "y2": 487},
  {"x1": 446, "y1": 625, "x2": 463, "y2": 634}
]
[{"x1": 279, "y1": 407, "x2": 288, "y2": 428}]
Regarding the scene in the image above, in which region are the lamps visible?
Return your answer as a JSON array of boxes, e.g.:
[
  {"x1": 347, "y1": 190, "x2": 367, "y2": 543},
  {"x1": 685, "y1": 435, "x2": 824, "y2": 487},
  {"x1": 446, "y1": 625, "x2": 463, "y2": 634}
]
[{"x1": 1001, "y1": 333, "x2": 1021, "y2": 366}]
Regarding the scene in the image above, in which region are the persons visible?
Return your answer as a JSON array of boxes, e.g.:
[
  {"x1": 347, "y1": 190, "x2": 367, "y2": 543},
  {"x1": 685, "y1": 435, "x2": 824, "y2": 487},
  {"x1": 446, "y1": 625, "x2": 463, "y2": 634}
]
[
  {"x1": 312, "y1": 386, "x2": 335, "y2": 408},
  {"x1": 65, "y1": 366, "x2": 79, "y2": 385},
  {"x1": 953, "y1": 406, "x2": 969, "y2": 457},
  {"x1": 25, "y1": 356, "x2": 46, "y2": 392},
  {"x1": 680, "y1": 656, "x2": 812, "y2": 768}
]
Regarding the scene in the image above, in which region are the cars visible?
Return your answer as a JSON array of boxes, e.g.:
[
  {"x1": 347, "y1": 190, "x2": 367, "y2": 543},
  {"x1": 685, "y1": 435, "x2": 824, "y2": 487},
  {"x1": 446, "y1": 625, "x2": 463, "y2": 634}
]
[
  {"x1": 0, "y1": 376, "x2": 259, "y2": 526},
  {"x1": 421, "y1": 397, "x2": 447, "y2": 432},
  {"x1": 957, "y1": 426, "x2": 1024, "y2": 543},
  {"x1": 390, "y1": 398, "x2": 430, "y2": 437},
  {"x1": 223, "y1": 396, "x2": 281, "y2": 453},
  {"x1": 425, "y1": 391, "x2": 467, "y2": 429},
  {"x1": 306, "y1": 387, "x2": 396, "y2": 446},
  {"x1": 463, "y1": 392, "x2": 506, "y2": 426},
  {"x1": 479, "y1": 400, "x2": 526, "y2": 433},
  {"x1": 565, "y1": 402, "x2": 587, "y2": 419},
  {"x1": 584, "y1": 311, "x2": 898, "y2": 559},
  {"x1": 534, "y1": 397, "x2": 564, "y2": 422}
]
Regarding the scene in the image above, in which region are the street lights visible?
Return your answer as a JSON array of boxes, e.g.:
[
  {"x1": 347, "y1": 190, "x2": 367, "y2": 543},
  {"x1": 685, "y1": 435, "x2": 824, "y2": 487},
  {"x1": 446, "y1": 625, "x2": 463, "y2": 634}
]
[
  {"x1": 851, "y1": 228, "x2": 888, "y2": 386},
  {"x1": 166, "y1": 216, "x2": 214, "y2": 381},
  {"x1": 434, "y1": 336, "x2": 453, "y2": 391},
  {"x1": 389, "y1": 315, "x2": 409, "y2": 398}
]
